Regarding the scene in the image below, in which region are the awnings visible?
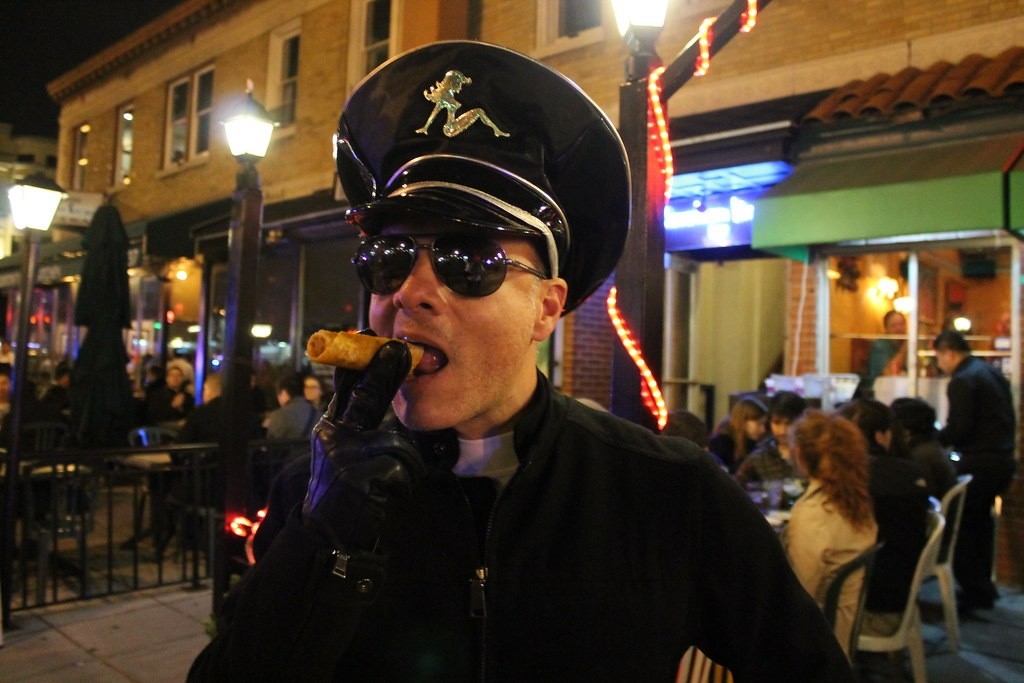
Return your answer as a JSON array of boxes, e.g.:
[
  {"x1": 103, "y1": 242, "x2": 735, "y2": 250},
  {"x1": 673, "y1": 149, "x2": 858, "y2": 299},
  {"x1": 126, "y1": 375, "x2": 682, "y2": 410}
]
[{"x1": 752, "y1": 130, "x2": 1024, "y2": 261}]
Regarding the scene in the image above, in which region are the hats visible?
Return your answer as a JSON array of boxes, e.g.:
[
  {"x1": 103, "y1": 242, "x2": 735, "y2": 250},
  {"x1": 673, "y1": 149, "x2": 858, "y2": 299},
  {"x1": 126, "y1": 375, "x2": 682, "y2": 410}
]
[{"x1": 336, "y1": 38, "x2": 634, "y2": 319}]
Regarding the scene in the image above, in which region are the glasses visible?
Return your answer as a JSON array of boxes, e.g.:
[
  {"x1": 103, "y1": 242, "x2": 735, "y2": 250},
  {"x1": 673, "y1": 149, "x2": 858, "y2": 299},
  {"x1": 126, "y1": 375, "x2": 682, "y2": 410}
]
[{"x1": 351, "y1": 226, "x2": 549, "y2": 298}]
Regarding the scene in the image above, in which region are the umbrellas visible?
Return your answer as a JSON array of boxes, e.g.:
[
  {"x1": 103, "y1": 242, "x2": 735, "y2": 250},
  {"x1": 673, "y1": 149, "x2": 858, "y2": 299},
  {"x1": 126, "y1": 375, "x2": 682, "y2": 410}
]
[{"x1": 74, "y1": 203, "x2": 132, "y2": 448}]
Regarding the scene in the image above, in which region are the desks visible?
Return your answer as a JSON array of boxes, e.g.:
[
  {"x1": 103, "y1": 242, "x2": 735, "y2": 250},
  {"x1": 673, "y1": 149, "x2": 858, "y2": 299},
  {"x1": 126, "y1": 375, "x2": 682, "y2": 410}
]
[
  {"x1": 109, "y1": 453, "x2": 172, "y2": 591},
  {"x1": 1, "y1": 463, "x2": 90, "y2": 578}
]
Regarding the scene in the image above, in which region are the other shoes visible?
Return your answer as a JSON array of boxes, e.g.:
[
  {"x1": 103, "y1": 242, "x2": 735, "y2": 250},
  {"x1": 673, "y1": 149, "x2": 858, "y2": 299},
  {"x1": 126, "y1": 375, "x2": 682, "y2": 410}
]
[{"x1": 954, "y1": 580, "x2": 1000, "y2": 612}]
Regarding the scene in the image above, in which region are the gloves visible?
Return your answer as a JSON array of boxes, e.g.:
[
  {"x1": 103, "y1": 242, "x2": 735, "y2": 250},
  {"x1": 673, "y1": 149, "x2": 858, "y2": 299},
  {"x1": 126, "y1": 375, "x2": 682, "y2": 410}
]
[{"x1": 297, "y1": 326, "x2": 439, "y2": 562}]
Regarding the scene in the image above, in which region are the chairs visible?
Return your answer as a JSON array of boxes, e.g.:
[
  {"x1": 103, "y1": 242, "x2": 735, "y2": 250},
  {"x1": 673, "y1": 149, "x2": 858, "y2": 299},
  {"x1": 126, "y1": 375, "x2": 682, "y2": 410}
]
[
  {"x1": 815, "y1": 474, "x2": 972, "y2": 683},
  {"x1": 0, "y1": 422, "x2": 180, "y2": 614}
]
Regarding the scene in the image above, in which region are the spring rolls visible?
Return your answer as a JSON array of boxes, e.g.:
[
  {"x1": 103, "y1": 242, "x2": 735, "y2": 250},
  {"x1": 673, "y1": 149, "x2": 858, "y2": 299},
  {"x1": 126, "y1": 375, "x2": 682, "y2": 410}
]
[{"x1": 305, "y1": 331, "x2": 423, "y2": 375}]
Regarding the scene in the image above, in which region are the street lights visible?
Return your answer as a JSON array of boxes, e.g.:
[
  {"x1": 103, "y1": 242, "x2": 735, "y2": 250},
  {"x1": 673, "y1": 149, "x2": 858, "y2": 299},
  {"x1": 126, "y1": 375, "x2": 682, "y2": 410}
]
[
  {"x1": 0, "y1": 171, "x2": 68, "y2": 629},
  {"x1": 606, "y1": 0, "x2": 665, "y2": 432},
  {"x1": 211, "y1": 79, "x2": 282, "y2": 618}
]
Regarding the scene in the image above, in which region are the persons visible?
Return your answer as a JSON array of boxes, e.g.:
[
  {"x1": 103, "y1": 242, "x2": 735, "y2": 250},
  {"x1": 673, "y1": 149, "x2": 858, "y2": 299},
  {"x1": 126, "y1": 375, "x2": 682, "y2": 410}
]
[
  {"x1": 934, "y1": 330, "x2": 1016, "y2": 610},
  {"x1": 0, "y1": 342, "x2": 329, "y2": 550},
  {"x1": 868, "y1": 311, "x2": 908, "y2": 376},
  {"x1": 663, "y1": 391, "x2": 960, "y2": 683},
  {"x1": 185, "y1": 39, "x2": 852, "y2": 683}
]
